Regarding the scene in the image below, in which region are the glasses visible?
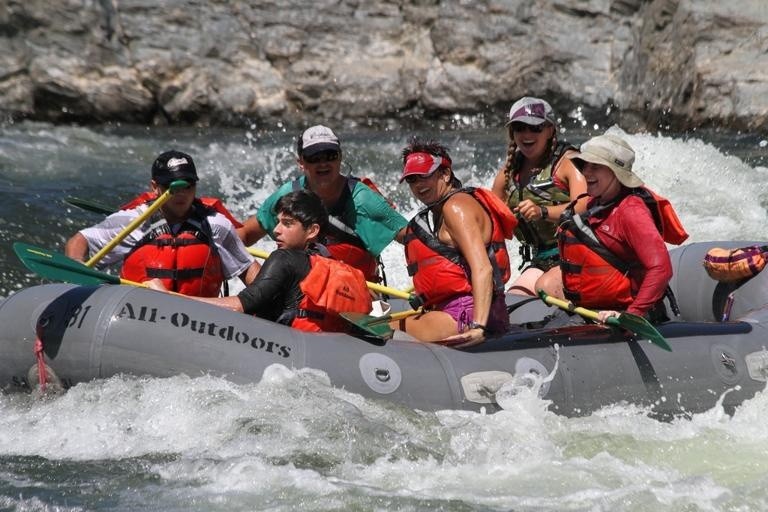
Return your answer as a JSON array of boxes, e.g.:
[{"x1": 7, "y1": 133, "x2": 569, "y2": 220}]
[
  {"x1": 405, "y1": 173, "x2": 434, "y2": 186},
  {"x1": 509, "y1": 122, "x2": 547, "y2": 134},
  {"x1": 306, "y1": 151, "x2": 340, "y2": 164}
]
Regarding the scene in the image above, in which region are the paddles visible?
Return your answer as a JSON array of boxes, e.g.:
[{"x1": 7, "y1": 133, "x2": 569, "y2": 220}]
[
  {"x1": 13, "y1": 240, "x2": 149, "y2": 287},
  {"x1": 537, "y1": 287, "x2": 673, "y2": 352},
  {"x1": 339, "y1": 309, "x2": 423, "y2": 342},
  {"x1": 65, "y1": 194, "x2": 414, "y2": 302}
]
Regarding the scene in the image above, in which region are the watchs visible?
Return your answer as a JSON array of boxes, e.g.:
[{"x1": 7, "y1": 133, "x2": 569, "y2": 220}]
[
  {"x1": 468, "y1": 320, "x2": 487, "y2": 331},
  {"x1": 536, "y1": 204, "x2": 548, "y2": 220}
]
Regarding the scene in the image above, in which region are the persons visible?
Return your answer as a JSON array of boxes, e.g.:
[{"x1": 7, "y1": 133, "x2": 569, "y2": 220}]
[
  {"x1": 556, "y1": 136, "x2": 673, "y2": 329},
  {"x1": 65, "y1": 151, "x2": 261, "y2": 292},
  {"x1": 492, "y1": 97, "x2": 593, "y2": 301},
  {"x1": 389, "y1": 135, "x2": 510, "y2": 346},
  {"x1": 170, "y1": 190, "x2": 332, "y2": 332},
  {"x1": 236, "y1": 125, "x2": 414, "y2": 260}
]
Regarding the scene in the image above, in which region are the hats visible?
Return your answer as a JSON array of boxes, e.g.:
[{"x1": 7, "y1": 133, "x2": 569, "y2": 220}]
[
  {"x1": 504, "y1": 96, "x2": 556, "y2": 128},
  {"x1": 396, "y1": 152, "x2": 451, "y2": 184},
  {"x1": 560, "y1": 124, "x2": 644, "y2": 189},
  {"x1": 150, "y1": 149, "x2": 199, "y2": 184},
  {"x1": 296, "y1": 124, "x2": 341, "y2": 160}
]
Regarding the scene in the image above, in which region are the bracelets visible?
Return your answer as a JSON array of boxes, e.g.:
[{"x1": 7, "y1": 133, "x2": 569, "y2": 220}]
[{"x1": 72, "y1": 257, "x2": 86, "y2": 262}]
